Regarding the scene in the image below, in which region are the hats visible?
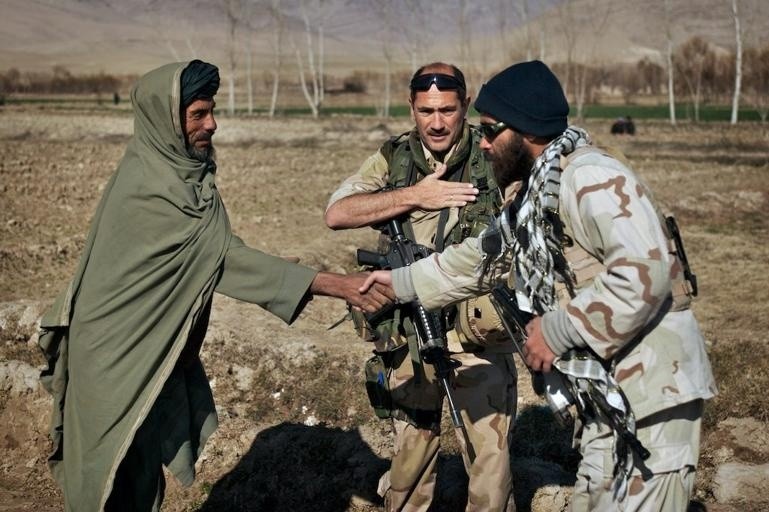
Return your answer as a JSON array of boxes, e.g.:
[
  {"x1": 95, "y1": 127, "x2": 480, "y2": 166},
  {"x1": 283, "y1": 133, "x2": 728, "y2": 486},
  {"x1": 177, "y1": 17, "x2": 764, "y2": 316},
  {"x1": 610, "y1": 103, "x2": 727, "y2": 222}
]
[{"x1": 474, "y1": 59, "x2": 571, "y2": 138}]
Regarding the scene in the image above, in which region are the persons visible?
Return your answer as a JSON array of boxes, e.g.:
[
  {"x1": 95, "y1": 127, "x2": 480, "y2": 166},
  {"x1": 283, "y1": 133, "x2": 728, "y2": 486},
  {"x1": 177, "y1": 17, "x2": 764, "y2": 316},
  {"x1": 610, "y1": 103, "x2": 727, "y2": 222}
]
[
  {"x1": 611, "y1": 114, "x2": 624, "y2": 133},
  {"x1": 345, "y1": 58, "x2": 721, "y2": 511},
  {"x1": 621, "y1": 115, "x2": 637, "y2": 134},
  {"x1": 320, "y1": 60, "x2": 526, "y2": 511},
  {"x1": 33, "y1": 57, "x2": 398, "y2": 511}
]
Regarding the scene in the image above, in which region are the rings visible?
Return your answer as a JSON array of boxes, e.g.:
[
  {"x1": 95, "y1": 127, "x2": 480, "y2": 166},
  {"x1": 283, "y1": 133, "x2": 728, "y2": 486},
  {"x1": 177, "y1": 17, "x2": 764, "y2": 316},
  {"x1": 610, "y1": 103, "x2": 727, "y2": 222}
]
[{"x1": 450, "y1": 194, "x2": 454, "y2": 201}]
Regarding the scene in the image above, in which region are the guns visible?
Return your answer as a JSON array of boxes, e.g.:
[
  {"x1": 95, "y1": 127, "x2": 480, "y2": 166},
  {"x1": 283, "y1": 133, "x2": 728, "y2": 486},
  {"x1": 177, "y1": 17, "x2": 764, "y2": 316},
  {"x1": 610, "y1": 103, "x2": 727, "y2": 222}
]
[
  {"x1": 355, "y1": 185, "x2": 465, "y2": 429},
  {"x1": 488, "y1": 285, "x2": 651, "y2": 459}
]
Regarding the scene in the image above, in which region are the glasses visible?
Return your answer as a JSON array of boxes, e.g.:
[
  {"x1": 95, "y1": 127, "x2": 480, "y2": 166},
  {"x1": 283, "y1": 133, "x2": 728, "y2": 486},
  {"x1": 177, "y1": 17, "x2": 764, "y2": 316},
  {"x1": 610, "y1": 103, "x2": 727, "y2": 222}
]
[
  {"x1": 477, "y1": 120, "x2": 507, "y2": 140},
  {"x1": 409, "y1": 72, "x2": 468, "y2": 94}
]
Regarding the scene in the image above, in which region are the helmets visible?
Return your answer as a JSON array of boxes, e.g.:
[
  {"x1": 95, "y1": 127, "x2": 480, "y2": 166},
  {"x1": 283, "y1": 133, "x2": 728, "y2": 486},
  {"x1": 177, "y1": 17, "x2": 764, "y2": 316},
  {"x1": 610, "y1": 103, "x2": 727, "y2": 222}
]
[{"x1": 459, "y1": 292, "x2": 513, "y2": 347}]
